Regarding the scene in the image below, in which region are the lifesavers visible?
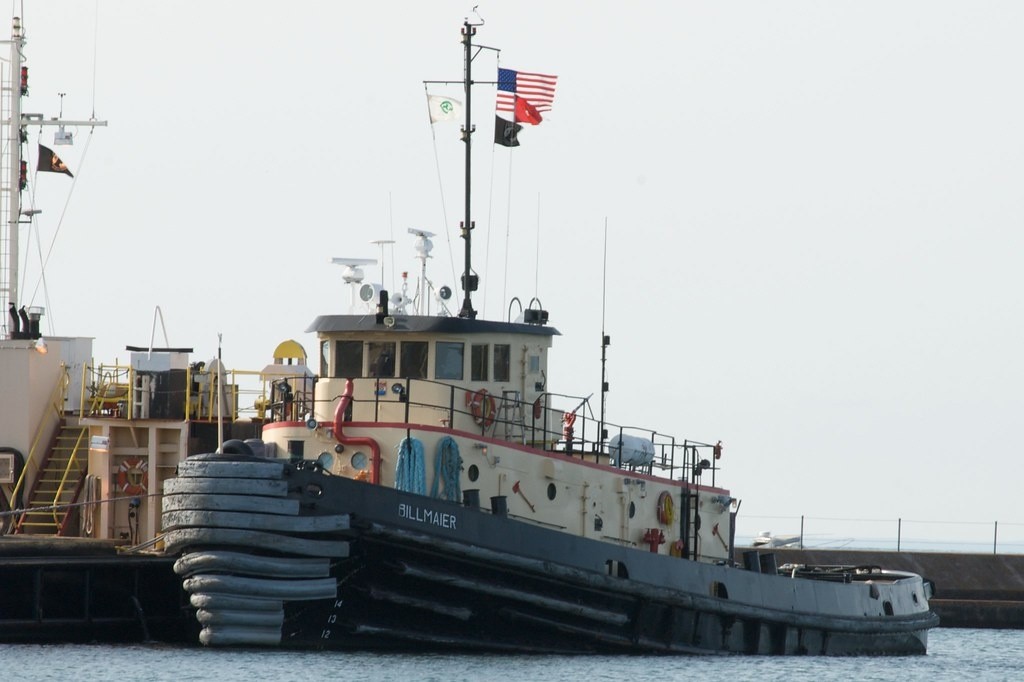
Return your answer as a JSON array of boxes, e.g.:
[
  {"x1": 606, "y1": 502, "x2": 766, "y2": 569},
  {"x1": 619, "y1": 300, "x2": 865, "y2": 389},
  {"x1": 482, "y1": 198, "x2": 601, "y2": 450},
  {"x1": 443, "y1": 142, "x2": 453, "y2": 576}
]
[
  {"x1": 469, "y1": 385, "x2": 499, "y2": 430},
  {"x1": 654, "y1": 487, "x2": 677, "y2": 528},
  {"x1": 114, "y1": 453, "x2": 151, "y2": 496}
]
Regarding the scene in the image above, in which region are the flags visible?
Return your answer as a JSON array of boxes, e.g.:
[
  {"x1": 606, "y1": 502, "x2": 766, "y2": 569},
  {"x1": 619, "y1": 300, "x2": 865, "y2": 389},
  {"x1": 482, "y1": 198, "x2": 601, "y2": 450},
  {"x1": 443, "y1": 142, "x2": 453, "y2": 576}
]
[
  {"x1": 496, "y1": 67, "x2": 558, "y2": 112},
  {"x1": 37, "y1": 143, "x2": 73, "y2": 177},
  {"x1": 514, "y1": 94, "x2": 543, "y2": 125},
  {"x1": 495, "y1": 115, "x2": 522, "y2": 147},
  {"x1": 427, "y1": 94, "x2": 462, "y2": 123}
]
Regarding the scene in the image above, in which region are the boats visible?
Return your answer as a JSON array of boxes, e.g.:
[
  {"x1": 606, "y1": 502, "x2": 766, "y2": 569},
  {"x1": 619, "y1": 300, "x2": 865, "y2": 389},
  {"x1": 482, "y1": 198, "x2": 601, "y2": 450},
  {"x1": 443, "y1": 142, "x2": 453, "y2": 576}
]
[{"x1": 1, "y1": 0, "x2": 944, "y2": 656}]
